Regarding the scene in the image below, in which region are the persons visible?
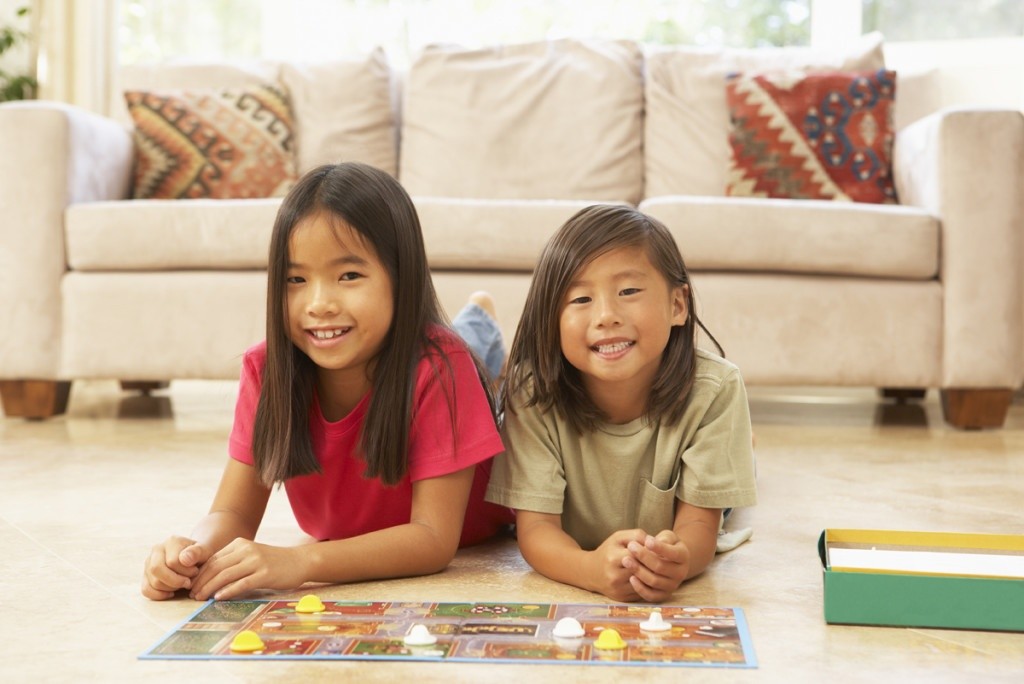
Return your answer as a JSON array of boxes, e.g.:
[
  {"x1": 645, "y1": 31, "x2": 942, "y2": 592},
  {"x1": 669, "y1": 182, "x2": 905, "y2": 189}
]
[
  {"x1": 140, "y1": 163, "x2": 519, "y2": 600},
  {"x1": 482, "y1": 204, "x2": 755, "y2": 604}
]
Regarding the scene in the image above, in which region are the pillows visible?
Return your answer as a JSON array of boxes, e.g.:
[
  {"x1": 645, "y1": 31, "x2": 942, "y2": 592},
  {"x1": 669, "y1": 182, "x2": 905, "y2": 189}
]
[
  {"x1": 720, "y1": 63, "x2": 901, "y2": 207},
  {"x1": 122, "y1": 66, "x2": 303, "y2": 199}
]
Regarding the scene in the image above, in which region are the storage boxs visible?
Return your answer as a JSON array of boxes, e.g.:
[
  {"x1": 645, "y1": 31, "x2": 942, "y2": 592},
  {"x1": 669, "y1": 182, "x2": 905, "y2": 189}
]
[{"x1": 817, "y1": 527, "x2": 1023, "y2": 632}]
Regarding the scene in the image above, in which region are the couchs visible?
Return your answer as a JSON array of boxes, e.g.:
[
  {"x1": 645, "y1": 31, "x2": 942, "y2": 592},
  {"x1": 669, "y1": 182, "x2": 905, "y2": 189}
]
[{"x1": 1, "y1": 33, "x2": 1024, "y2": 423}]
